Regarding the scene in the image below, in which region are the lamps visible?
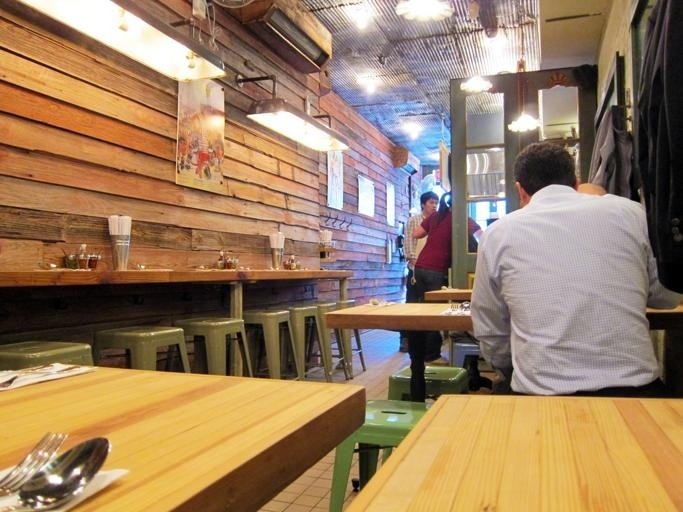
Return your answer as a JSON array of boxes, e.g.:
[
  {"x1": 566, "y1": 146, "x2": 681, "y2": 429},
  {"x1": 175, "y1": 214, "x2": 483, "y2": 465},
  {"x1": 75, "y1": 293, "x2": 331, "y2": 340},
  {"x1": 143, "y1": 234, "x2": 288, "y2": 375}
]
[
  {"x1": 2, "y1": 0, "x2": 225, "y2": 82},
  {"x1": 236, "y1": 73, "x2": 351, "y2": 153}
]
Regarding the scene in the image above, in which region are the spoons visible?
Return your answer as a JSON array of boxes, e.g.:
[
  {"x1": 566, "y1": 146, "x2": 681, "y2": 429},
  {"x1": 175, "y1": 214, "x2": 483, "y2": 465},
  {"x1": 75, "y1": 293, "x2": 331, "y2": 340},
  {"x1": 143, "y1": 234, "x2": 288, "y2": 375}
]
[
  {"x1": 0, "y1": 437, "x2": 113, "y2": 512},
  {"x1": 458, "y1": 301, "x2": 470, "y2": 317}
]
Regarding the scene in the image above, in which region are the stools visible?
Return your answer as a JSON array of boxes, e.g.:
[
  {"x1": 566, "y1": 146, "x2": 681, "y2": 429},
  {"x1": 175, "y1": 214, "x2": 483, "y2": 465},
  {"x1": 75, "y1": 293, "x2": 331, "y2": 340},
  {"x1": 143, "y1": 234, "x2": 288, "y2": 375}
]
[
  {"x1": 329, "y1": 399, "x2": 429, "y2": 512},
  {"x1": 0, "y1": 339, "x2": 94, "y2": 371},
  {"x1": 381, "y1": 364, "x2": 470, "y2": 469},
  {"x1": 93, "y1": 299, "x2": 367, "y2": 383},
  {"x1": 451, "y1": 341, "x2": 487, "y2": 369}
]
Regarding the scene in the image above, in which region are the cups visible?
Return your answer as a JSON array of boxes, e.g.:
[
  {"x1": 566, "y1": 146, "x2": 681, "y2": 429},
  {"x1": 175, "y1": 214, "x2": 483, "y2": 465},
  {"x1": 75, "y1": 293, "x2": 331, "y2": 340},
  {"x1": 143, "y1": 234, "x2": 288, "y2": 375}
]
[
  {"x1": 109, "y1": 234, "x2": 130, "y2": 270},
  {"x1": 271, "y1": 248, "x2": 282, "y2": 271}
]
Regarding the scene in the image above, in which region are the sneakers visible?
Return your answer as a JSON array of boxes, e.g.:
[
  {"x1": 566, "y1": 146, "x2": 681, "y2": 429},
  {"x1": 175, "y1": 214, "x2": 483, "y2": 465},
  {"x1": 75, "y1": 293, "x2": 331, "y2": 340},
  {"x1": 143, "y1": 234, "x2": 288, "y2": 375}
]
[
  {"x1": 425, "y1": 356, "x2": 448, "y2": 365},
  {"x1": 398, "y1": 338, "x2": 408, "y2": 352}
]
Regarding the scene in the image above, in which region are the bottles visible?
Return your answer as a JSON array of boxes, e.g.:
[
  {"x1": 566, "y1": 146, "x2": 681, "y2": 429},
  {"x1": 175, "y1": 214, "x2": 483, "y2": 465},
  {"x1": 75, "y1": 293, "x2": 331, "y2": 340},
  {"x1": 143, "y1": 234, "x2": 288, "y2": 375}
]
[
  {"x1": 64, "y1": 249, "x2": 100, "y2": 269},
  {"x1": 216, "y1": 247, "x2": 240, "y2": 269},
  {"x1": 283, "y1": 254, "x2": 302, "y2": 270}
]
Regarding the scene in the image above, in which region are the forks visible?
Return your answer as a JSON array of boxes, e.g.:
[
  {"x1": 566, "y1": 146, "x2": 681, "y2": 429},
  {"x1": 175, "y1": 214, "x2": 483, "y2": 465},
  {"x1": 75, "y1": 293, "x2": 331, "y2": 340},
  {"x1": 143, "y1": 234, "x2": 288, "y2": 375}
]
[
  {"x1": 444, "y1": 304, "x2": 459, "y2": 315},
  {"x1": 0, "y1": 432, "x2": 68, "y2": 497},
  {"x1": 0, "y1": 365, "x2": 81, "y2": 391}
]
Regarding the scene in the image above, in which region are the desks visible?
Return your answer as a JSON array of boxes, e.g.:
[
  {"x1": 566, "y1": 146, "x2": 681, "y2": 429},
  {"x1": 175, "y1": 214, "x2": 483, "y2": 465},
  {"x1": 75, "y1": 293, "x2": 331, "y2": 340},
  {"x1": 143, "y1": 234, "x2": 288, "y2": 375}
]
[
  {"x1": 0, "y1": 269, "x2": 355, "y2": 377},
  {"x1": 0, "y1": 363, "x2": 365, "y2": 512},
  {"x1": 324, "y1": 298, "x2": 682, "y2": 404},
  {"x1": 340, "y1": 394, "x2": 683, "y2": 512},
  {"x1": 424, "y1": 288, "x2": 472, "y2": 304}
]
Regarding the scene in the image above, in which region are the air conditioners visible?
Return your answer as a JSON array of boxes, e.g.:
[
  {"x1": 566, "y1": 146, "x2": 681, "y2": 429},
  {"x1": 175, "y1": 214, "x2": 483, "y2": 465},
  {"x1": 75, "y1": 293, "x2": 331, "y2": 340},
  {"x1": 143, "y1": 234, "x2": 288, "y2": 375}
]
[
  {"x1": 229, "y1": 0, "x2": 333, "y2": 74},
  {"x1": 393, "y1": 148, "x2": 420, "y2": 175}
]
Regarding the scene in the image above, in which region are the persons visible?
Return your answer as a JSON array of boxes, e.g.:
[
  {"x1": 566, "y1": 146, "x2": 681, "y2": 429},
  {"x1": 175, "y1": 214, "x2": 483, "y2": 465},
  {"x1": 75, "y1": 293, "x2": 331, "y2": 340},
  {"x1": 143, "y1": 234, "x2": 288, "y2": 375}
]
[
  {"x1": 405, "y1": 191, "x2": 484, "y2": 362},
  {"x1": 577, "y1": 183, "x2": 607, "y2": 196},
  {"x1": 399, "y1": 192, "x2": 439, "y2": 353},
  {"x1": 469, "y1": 141, "x2": 683, "y2": 398}
]
